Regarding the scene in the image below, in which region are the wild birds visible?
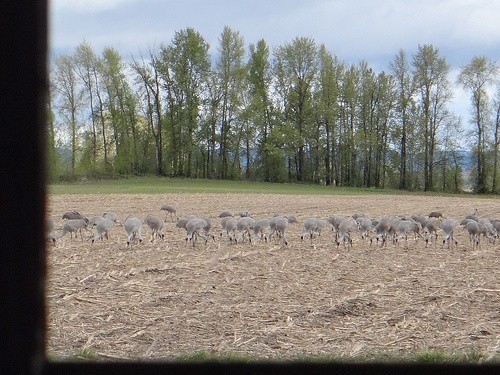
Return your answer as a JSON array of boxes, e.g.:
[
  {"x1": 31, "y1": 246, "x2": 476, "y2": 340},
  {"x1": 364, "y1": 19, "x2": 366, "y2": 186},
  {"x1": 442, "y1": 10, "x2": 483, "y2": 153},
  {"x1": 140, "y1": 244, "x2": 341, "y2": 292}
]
[{"x1": 47, "y1": 205, "x2": 499, "y2": 247}]
